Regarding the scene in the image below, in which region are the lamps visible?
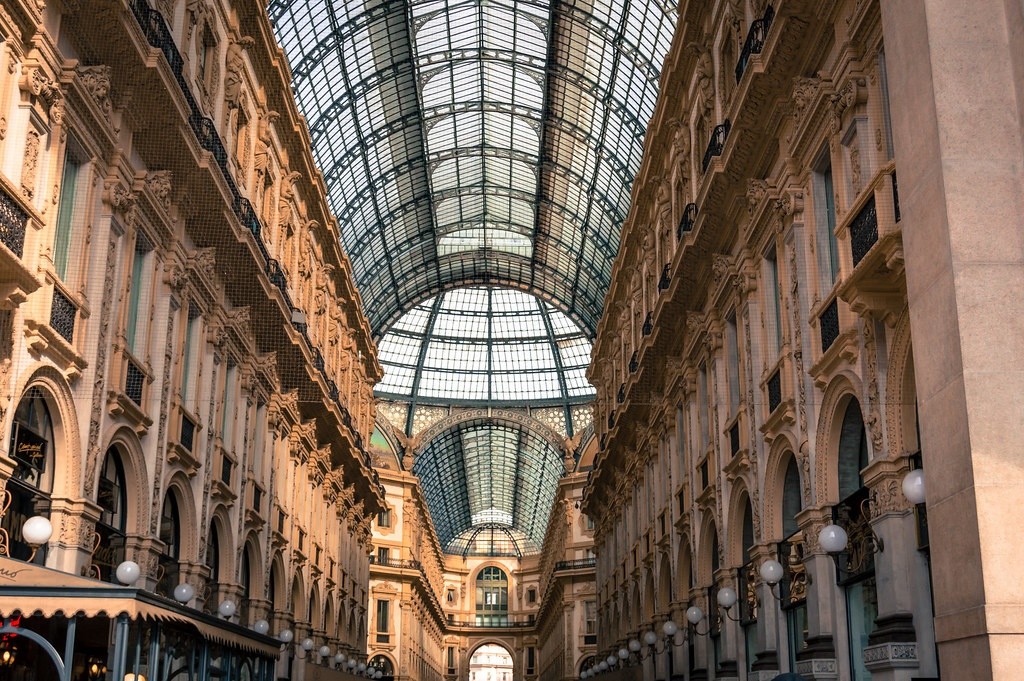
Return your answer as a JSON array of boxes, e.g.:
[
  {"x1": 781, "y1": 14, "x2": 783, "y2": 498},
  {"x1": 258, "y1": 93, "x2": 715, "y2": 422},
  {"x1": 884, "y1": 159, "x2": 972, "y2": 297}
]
[
  {"x1": 580, "y1": 467, "x2": 928, "y2": 680},
  {"x1": 21, "y1": 515, "x2": 384, "y2": 679}
]
